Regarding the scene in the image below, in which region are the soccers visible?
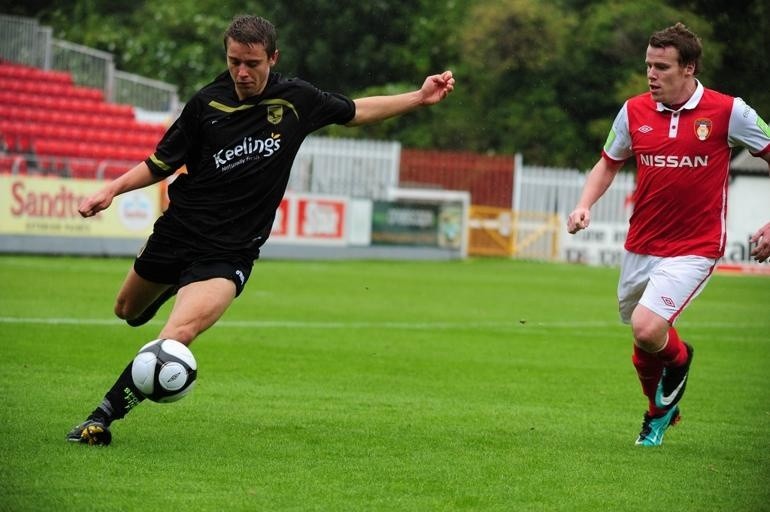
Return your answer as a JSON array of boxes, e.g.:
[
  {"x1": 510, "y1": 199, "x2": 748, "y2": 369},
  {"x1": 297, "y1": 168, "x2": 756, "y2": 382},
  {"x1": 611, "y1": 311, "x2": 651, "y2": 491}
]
[{"x1": 132, "y1": 338, "x2": 198, "y2": 404}]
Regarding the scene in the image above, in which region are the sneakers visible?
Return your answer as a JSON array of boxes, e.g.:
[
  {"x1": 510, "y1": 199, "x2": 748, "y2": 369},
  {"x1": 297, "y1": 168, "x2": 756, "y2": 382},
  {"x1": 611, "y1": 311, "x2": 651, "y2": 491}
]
[
  {"x1": 634, "y1": 402, "x2": 682, "y2": 448},
  {"x1": 655, "y1": 341, "x2": 694, "y2": 409},
  {"x1": 66, "y1": 417, "x2": 112, "y2": 446}
]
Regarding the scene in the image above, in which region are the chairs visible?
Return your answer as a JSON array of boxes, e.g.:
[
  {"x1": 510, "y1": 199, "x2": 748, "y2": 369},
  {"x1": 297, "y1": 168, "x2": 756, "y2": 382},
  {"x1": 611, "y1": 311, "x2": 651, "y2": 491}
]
[{"x1": 0, "y1": 61, "x2": 169, "y2": 178}]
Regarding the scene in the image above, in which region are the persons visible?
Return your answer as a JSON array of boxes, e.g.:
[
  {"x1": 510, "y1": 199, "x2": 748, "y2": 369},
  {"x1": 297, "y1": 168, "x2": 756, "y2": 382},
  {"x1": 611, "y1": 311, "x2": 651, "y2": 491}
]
[
  {"x1": 61, "y1": 11, "x2": 458, "y2": 446},
  {"x1": 565, "y1": 18, "x2": 769, "y2": 448}
]
